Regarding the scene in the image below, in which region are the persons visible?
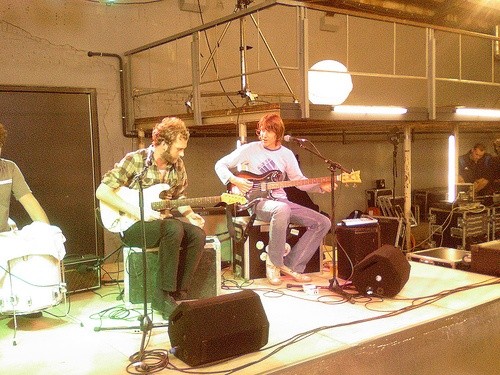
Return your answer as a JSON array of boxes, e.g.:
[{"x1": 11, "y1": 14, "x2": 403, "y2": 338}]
[
  {"x1": 215, "y1": 113, "x2": 338, "y2": 284},
  {"x1": 0, "y1": 125, "x2": 50, "y2": 319},
  {"x1": 459, "y1": 139, "x2": 500, "y2": 206},
  {"x1": 94, "y1": 116, "x2": 206, "y2": 317}
]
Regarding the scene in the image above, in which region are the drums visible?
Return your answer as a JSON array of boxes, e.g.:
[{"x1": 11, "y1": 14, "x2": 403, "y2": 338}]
[{"x1": 0, "y1": 233, "x2": 63, "y2": 315}]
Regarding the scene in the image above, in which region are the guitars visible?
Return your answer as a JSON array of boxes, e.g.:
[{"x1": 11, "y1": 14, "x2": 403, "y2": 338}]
[
  {"x1": 225, "y1": 170, "x2": 363, "y2": 209},
  {"x1": 98, "y1": 181, "x2": 249, "y2": 235}
]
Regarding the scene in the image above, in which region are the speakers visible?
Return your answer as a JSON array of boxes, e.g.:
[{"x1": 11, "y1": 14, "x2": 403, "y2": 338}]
[
  {"x1": 168, "y1": 288, "x2": 270, "y2": 367},
  {"x1": 336, "y1": 226, "x2": 380, "y2": 280},
  {"x1": 350, "y1": 243, "x2": 410, "y2": 300},
  {"x1": 61, "y1": 253, "x2": 102, "y2": 294}
]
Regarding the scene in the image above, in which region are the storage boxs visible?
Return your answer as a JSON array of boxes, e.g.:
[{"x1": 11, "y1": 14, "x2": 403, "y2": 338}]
[
  {"x1": 122, "y1": 235, "x2": 223, "y2": 310},
  {"x1": 365, "y1": 183, "x2": 500, "y2": 281},
  {"x1": 228, "y1": 216, "x2": 325, "y2": 280}
]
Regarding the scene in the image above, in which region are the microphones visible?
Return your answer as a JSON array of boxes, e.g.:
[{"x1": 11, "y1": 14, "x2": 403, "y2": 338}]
[
  {"x1": 76, "y1": 265, "x2": 89, "y2": 274},
  {"x1": 147, "y1": 144, "x2": 155, "y2": 164},
  {"x1": 283, "y1": 135, "x2": 308, "y2": 143}
]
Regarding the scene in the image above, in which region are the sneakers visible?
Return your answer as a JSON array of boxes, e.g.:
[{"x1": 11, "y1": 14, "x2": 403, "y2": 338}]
[
  {"x1": 266, "y1": 254, "x2": 282, "y2": 285},
  {"x1": 151, "y1": 292, "x2": 178, "y2": 315},
  {"x1": 281, "y1": 264, "x2": 311, "y2": 283}
]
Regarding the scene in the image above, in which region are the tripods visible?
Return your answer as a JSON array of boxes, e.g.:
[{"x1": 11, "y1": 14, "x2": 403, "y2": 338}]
[
  {"x1": 94, "y1": 161, "x2": 171, "y2": 361},
  {"x1": 287, "y1": 143, "x2": 356, "y2": 305}
]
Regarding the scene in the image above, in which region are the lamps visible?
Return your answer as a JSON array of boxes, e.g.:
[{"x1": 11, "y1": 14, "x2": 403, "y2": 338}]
[
  {"x1": 447, "y1": 131, "x2": 455, "y2": 204},
  {"x1": 329, "y1": 101, "x2": 409, "y2": 118},
  {"x1": 449, "y1": 106, "x2": 500, "y2": 121}
]
